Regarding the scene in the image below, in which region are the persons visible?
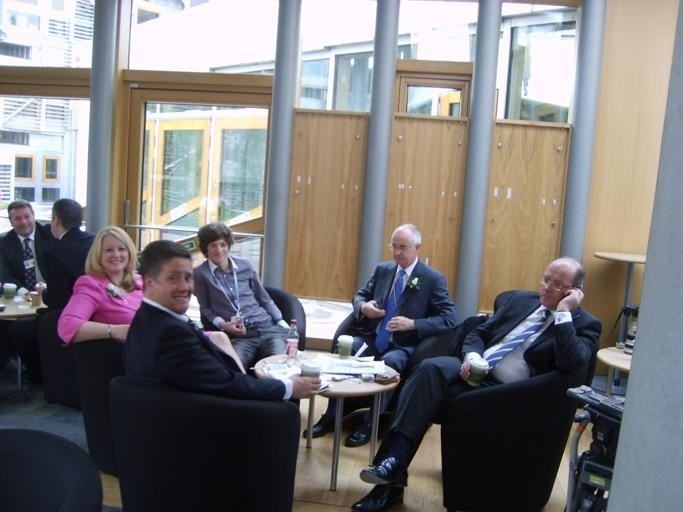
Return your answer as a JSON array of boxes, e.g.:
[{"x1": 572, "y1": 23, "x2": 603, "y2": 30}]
[
  {"x1": 0, "y1": 200, "x2": 59, "y2": 371},
  {"x1": 303, "y1": 221, "x2": 458, "y2": 446},
  {"x1": 9, "y1": 198, "x2": 98, "y2": 384},
  {"x1": 123, "y1": 239, "x2": 321, "y2": 402},
  {"x1": 193, "y1": 223, "x2": 300, "y2": 371},
  {"x1": 350, "y1": 255, "x2": 603, "y2": 512},
  {"x1": 56, "y1": 224, "x2": 247, "y2": 374}
]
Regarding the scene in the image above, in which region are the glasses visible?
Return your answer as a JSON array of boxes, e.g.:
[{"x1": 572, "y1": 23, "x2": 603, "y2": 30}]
[{"x1": 540, "y1": 277, "x2": 569, "y2": 291}]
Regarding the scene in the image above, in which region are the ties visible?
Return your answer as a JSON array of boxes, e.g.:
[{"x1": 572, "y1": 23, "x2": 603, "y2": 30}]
[
  {"x1": 486, "y1": 309, "x2": 550, "y2": 375},
  {"x1": 22, "y1": 237, "x2": 37, "y2": 292},
  {"x1": 374, "y1": 269, "x2": 407, "y2": 354}
]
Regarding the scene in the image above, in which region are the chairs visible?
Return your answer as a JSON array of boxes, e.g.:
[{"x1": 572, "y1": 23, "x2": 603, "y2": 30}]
[
  {"x1": 438, "y1": 364, "x2": 592, "y2": 511},
  {"x1": 1, "y1": 425, "x2": 111, "y2": 512},
  {"x1": 34, "y1": 305, "x2": 128, "y2": 420},
  {"x1": 330, "y1": 311, "x2": 442, "y2": 435},
  {"x1": 110, "y1": 376, "x2": 302, "y2": 512},
  {"x1": 198, "y1": 285, "x2": 310, "y2": 352}
]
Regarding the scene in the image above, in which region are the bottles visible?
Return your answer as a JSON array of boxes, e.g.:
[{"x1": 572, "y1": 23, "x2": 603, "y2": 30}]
[
  {"x1": 579, "y1": 485, "x2": 600, "y2": 512},
  {"x1": 284, "y1": 319, "x2": 299, "y2": 358}
]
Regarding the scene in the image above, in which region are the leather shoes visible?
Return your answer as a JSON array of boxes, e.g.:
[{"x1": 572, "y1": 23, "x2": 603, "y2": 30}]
[
  {"x1": 352, "y1": 484, "x2": 403, "y2": 512},
  {"x1": 360, "y1": 458, "x2": 409, "y2": 487},
  {"x1": 302, "y1": 414, "x2": 336, "y2": 437},
  {"x1": 346, "y1": 426, "x2": 373, "y2": 446}
]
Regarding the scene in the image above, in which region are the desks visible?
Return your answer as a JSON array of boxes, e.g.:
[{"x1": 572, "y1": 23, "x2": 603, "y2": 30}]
[
  {"x1": 594, "y1": 345, "x2": 634, "y2": 401},
  {"x1": 1, "y1": 287, "x2": 48, "y2": 394},
  {"x1": 252, "y1": 348, "x2": 401, "y2": 494},
  {"x1": 588, "y1": 248, "x2": 648, "y2": 344}
]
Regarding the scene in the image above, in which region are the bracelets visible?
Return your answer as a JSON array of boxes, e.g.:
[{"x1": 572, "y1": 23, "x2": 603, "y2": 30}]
[{"x1": 108, "y1": 324, "x2": 113, "y2": 340}]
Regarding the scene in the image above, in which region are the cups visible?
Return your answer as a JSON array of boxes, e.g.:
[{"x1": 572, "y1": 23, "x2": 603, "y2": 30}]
[
  {"x1": 300, "y1": 360, "x2": 321, "y2": 378},
  {"x1": 29, "y1": 291, "x2": 41, "y2": 306},
  {"x1": 3, "y1": 283, "x2": 16, "y2": 298},
  {"x1": 337, "y1": 334, "x2": 353, "y2": 358},
  {"x1": 466, "y1": 358, "x2": 489, "y2": 387}
]
